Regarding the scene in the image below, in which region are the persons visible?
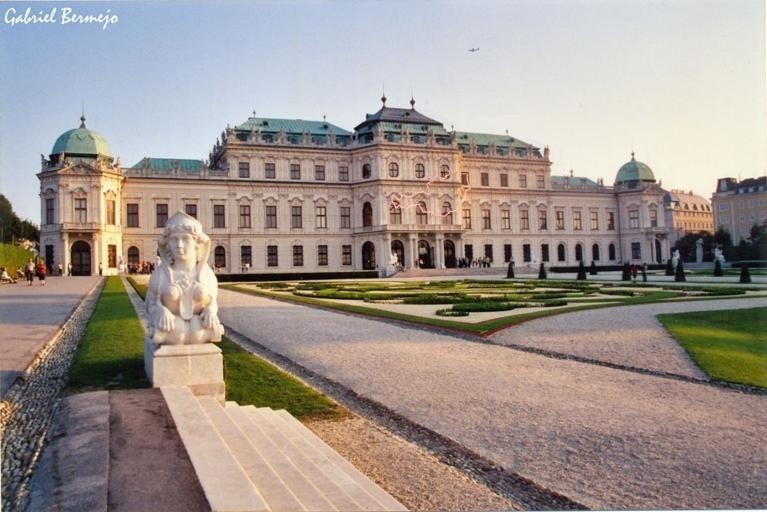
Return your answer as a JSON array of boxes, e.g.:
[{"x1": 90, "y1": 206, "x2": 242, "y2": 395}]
[
  {"x1": 367, "y1": 256, "x2": 372, "y2": 271},
  {"x1": 457, "y1": 256, "x2": 491, "y2": 268},
  {"x1": 631, "y1": 262, "x2": 648, "y2": 282},
  {"x1": 0, "y1": 258, "x2": 46, "y2": 286},
  {"x1": 510, "y1": 256, "x2": 515, "y2": 267},
  {"x1": 240, "y1": 260, "x2": 249, "y2": 272},
  {"x1": 397, "y1": 256, "x2": 424, "y2": 269},
  {"x1": 144, "y1": 211, "x2": 222, "y2": 345},
  {"x1": 124, "y1": 262, "x2": 154, "y2": 275},
  {"x1": 211, "y1": 263, "x2": 221, "y2": 273},
  {"x1": 99, "y1": 262, "x2": 103, "y2": 276},
  {"x1": 58, "y1": 263, "x2": 72, "y2": 277}
]
